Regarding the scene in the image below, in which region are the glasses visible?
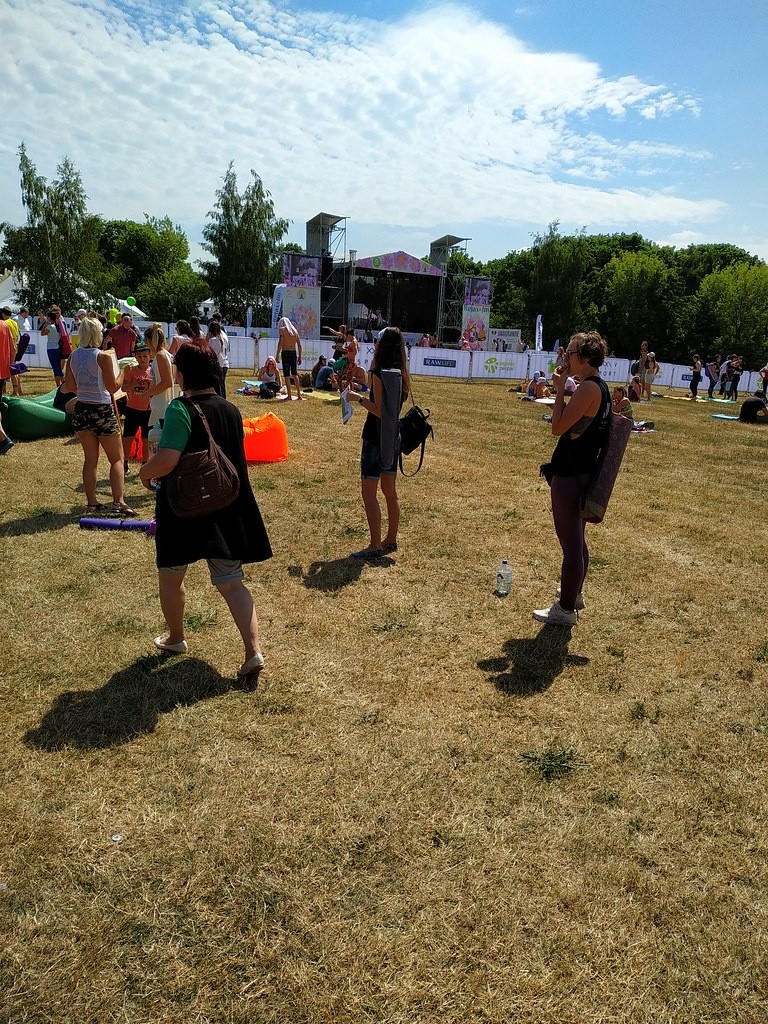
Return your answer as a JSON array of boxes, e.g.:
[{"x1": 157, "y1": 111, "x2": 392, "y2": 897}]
[
  {"x1": 562, "y1": 351, "x2": 578, "y2": 358},
  {"x1": 269, "y1": 364, "x2": 274, "y2": 365}
]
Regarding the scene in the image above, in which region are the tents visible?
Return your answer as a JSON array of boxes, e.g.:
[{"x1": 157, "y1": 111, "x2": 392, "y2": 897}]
[
  {"x1": 320, "y1": 250, "x2": 447, "y2": 347},
  {"x1": 0, "y1": 260, "x2": 272, "y2": 327}
]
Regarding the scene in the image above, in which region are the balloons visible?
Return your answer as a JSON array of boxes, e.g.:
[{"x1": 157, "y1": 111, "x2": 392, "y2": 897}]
[{"x1": 127, "y1": 297, "x2": 136, "y2": 306}]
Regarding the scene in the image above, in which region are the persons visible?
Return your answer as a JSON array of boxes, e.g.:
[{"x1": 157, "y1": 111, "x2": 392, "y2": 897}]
[
  {"x1": 250, "y1": 332, "x2": 263, "y2": 343},
  {"x1": 258, "y1": 356, "x2": 282, "y2": 398},
  {"x1": 419, "y1": 330, "x2": 507, "y2": 351},
  {"x1": 275, "y1": 317, "x2": 302, "y2": 400},
  {"x1": 312, "y1": 306, "x2": 383, "y2": 392},
  {"x1": 739, "y1": 390, "x2": 768, "y2": 424},
  {"x1": 345, "y1": 327, "x2": 410, "y2": 558},
  {"x1": 533, "y1": 331, "x2": 613, "y2": 624},
  {"x1": 66, "y1": 317, "x2": 139, "y2": 515},
  {"x1": 515, "y1": 339, "x2": 659, "y2": 424},
  {"x1": 140, "y1": 340, "x2": 273, "y2": 674},
  {"x1": 0, "y1": 305, "x2": 230, "y2": 475},
  {"x1": 464, "y1": 324, "x2": 487, "y2": 341},
  {"x1": 705, "y1": 354, "x2": 768, "y2": 401},
  {"x1": 690, "y1": 354, "x2": 701, "y2": 397}
]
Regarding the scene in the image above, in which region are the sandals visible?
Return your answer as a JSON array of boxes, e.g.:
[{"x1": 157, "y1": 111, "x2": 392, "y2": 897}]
[
  {"x1": 85, "y1": 503, "x2": 108, "y2": 516},
  {"x1": 111, "y1": 501, "x2": 139, "y2": 517}
]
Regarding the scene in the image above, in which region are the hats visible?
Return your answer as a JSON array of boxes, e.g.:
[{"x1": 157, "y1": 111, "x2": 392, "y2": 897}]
[
  {"x1": 75, "y1": 308, "x2": 86, "y2": 316},
  {"x1": 647, "y1": 352, "x2": 655, "y2": 358},
  {"x1": 0, "y1": 306, "x2": 12, "y2": 313},
  {"x1": 328, "y1": 358, "x2": 336, "y2": 365},
  {"x1": 536, "y1": 377, "x2": 546, "y2": 385},
  {"x1": 131, "y1": 342, "x2": 150, "y2": 353}
]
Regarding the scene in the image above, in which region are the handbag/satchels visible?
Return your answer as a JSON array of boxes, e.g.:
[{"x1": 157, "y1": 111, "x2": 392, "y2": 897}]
[
  {"x1": 166, "y1": 396, "x2": 240, "y2": 516},
  {"x1": 397, "y1": 406, "x2": 435, "y2": 477}
]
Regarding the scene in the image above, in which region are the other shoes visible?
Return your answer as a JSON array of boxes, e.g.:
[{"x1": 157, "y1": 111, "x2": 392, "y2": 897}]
[
  {"x1": 236, "y1": 653, "x2": 264, "y2": 676},
  {"x1": 0, "y1": 437, "x2": 14, "y2": 455},
  {"x1": 533, "y1": 602, "x2": 577, "y2": 627},
  {"x1": 153, "y1": 635, "x2": 188, "y2": 653},
  {"x1": 352, "y1": 540, "x2": 397, "y2": 560},
  {"x1": 556, "y1": 588, "x2": 585, "y2": 608}
]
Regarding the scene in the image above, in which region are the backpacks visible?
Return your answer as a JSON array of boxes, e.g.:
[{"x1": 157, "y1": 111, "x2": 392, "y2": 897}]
[
  {"x1": 83, "y1": 317, "x2": 103, "y2": 347},
  {"x1": 631, "y1": 359, "x2": 639, "y2": 376},
  {"x1": 260, "y1": 382, "x2": 281, "y2": 398},
  {"x1": 290, "y1": 373, "x2": 312, "y2": 389}
]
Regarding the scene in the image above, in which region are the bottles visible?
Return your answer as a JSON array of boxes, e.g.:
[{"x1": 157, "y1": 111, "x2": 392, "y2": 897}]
[
  {"x1": 497, "y1": 560, "x2": 511, "y2": 593},
  {"x1": 148, "y1": 421, "x2": 163, "y2": 489}
]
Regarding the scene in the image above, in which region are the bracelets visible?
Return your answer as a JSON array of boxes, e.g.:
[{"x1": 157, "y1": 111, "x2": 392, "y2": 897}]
[{"x1": 358, "y1": 396, "x2": 366, "y2": 404}]
